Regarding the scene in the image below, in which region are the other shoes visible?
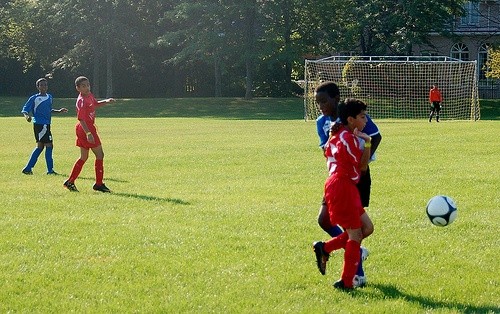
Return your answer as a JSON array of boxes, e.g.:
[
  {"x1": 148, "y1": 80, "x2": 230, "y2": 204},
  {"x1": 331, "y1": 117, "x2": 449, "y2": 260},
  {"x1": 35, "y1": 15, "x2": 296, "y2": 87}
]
[
  {"x1": 47, "y1": 171, "x2": 56, "y2": 174},
  {"x1": 22, "y1": 169, "x2": 32, "y2": 175}
]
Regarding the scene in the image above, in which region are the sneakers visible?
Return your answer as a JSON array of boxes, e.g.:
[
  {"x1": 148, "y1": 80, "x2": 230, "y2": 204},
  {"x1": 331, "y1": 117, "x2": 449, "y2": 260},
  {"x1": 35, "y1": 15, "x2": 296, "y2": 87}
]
[
  {"x1": 360, "y1": 248, "x2": 368, "y2": 261},
  {"x1": 333, "y1": 279, "x2": 352, "y2": 291},
  {"x1": 64, "y1": 180, "x2": 78, "y2": 191},
  {"x1": 93, "y1": 183, "x2": 110, "y2": 193},
  {"x1": 314, "y1": 241, "x2": 330, "y2": 275},
  {"x1": 352, "y1": 273, "x2": 365, "y2": 287}
]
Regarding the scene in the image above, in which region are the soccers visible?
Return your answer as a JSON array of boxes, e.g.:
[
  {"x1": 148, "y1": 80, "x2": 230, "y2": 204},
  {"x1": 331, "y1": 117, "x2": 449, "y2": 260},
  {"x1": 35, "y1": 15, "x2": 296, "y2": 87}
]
[{"x1": 425, "y1": 195, "x2": 458, "y2": 226}]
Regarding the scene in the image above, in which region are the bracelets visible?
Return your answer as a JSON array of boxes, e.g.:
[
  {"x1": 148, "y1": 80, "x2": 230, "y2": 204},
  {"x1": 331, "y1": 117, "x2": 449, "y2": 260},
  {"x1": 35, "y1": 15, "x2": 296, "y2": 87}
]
[
  {"x1": 364, "y1": 143, "x2": 371, "y2": 147},
  {"x1": 86, "y1": 131, "x2": 91, "y2": 135}
]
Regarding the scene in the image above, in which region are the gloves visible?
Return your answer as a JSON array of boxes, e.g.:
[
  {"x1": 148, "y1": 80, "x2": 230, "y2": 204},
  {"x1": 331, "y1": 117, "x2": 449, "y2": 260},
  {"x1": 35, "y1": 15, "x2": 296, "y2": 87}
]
[
  {"x1": 440, "y1": 104, "x2": 442, "y2": 108},
  {"x1": 431, "y1": 103, "x2": 434, "y2": 108}
]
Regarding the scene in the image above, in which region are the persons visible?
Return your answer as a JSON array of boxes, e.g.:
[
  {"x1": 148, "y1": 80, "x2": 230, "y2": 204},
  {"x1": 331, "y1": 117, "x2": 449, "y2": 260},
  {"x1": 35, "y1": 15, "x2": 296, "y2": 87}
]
[
  {"x1": 22, "y1": 78, "x2": 68, "y2": 175},
  {"x1": 314, "y1": 82, "x2": 382, "y2": 286},
  {"x1": 64, "y1": 76, "x2": 113, "y2": 193},
  {"x1": 429, "y1": 83, "x2": 442, "y2": 122},
  {"x1": 314, "y1": 97, "x2": 374, "y2": 294}
]
